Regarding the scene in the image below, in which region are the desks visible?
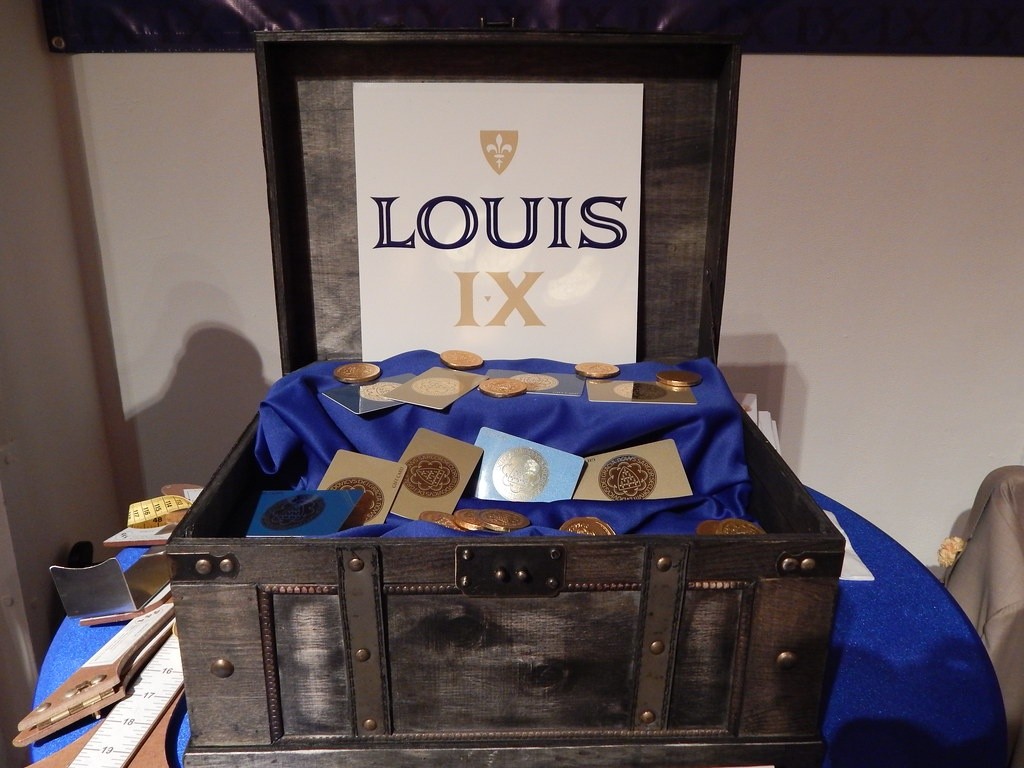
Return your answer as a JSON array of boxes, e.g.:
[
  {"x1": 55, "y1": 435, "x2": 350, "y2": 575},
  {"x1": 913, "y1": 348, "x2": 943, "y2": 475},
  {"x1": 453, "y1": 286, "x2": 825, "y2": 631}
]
[{"x1": 24, "y1": 477, "x2": 1011, "y2": 768}]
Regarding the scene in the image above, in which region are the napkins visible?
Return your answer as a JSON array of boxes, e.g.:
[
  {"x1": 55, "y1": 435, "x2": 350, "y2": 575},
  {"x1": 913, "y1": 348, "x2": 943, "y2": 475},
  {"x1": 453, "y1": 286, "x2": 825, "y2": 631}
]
[{"x1": 823, "y1": 509, "x2": 876, "y2": 582}]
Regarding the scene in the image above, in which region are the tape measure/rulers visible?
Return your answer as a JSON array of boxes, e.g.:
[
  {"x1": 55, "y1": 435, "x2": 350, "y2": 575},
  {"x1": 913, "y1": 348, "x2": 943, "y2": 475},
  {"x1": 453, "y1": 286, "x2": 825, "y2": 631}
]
[
  {"x1": 125, "y1": 495, "x2": 192, "y2": 527},
  {"x1": 69, "y1": 634, "x2": 186, "y2": 768}
]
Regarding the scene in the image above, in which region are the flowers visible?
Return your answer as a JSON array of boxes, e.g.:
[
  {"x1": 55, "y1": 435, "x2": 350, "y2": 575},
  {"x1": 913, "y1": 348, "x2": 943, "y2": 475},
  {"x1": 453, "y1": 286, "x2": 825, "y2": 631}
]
[{"x1": 936, "y1": 534, "x2": 966, "y2": 568}]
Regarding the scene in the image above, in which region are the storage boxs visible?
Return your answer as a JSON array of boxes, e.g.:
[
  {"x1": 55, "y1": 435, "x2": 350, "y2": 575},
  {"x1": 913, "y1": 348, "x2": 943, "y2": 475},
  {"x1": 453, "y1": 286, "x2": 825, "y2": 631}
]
[{"x1": 156, "y1": 376, "x2": 846, "y2": 768}]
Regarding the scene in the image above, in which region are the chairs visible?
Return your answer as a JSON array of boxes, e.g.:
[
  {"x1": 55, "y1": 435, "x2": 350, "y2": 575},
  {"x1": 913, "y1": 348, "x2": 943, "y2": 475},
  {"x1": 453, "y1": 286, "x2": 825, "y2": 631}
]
[{"x1": 936, "y1": 464, "x2": 1024, "y2": 722}]
